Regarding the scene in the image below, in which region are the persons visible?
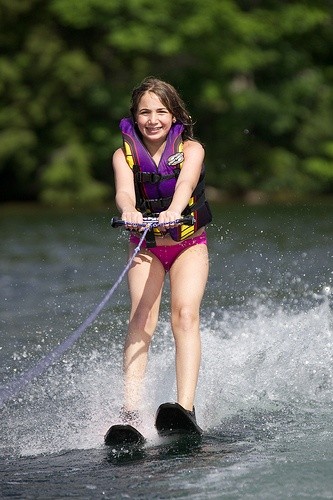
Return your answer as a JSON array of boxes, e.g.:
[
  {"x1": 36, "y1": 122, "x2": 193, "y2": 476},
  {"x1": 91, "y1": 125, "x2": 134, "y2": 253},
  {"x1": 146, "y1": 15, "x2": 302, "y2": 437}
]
[{"x1": 109, "y1": 75, "x2": 212, "y2": 437}]
